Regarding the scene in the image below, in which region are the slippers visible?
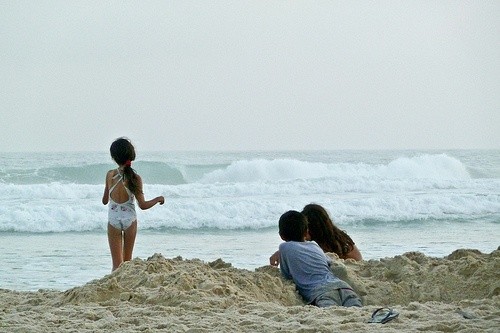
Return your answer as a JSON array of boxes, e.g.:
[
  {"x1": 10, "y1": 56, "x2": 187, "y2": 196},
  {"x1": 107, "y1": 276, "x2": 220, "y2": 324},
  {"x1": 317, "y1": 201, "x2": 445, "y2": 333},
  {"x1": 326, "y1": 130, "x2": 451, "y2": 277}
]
[{"x1": 367, "y1": 306, "x2": 399, "y2": 324}]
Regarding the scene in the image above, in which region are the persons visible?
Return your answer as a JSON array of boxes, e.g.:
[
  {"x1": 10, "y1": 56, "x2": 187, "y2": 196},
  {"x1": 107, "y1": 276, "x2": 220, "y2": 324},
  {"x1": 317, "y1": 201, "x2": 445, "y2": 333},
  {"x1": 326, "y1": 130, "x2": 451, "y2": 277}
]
[
  {"x1": 268, "y1": 204, "x2": 362, "y2": 266},
  {"x1": 102, "y1": 137, "x2": 167, "y2": 273},
  {"x1": 277, "y1": 209, "x2": 364, "y2": 308}
]
